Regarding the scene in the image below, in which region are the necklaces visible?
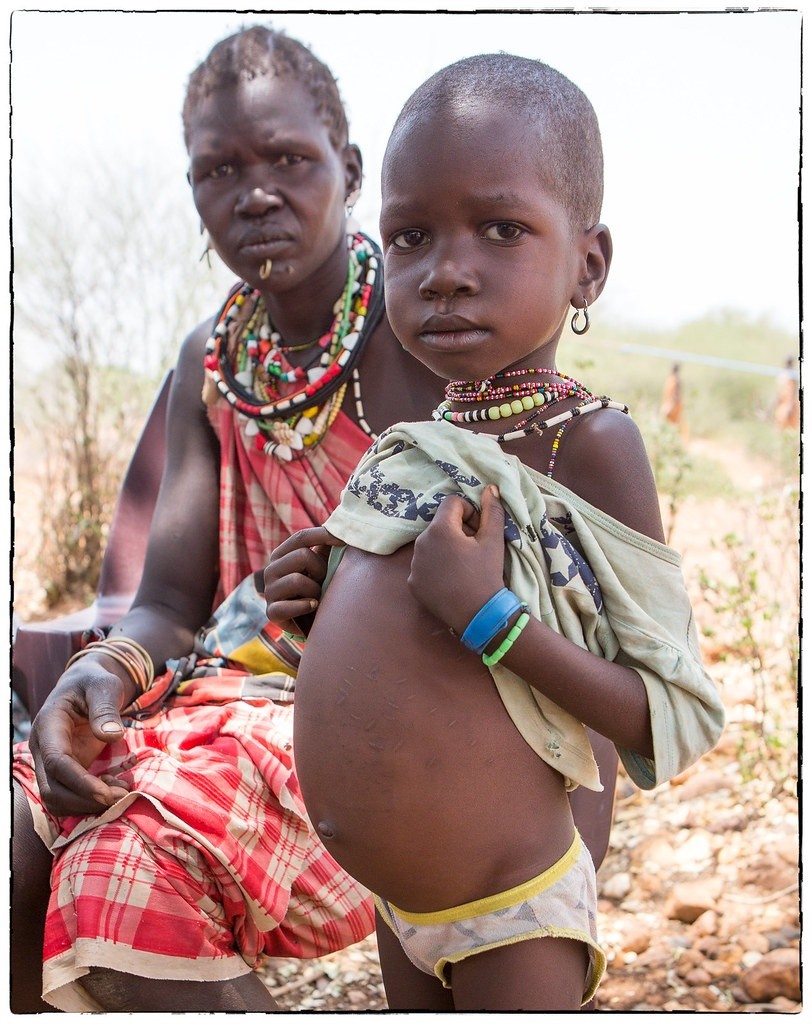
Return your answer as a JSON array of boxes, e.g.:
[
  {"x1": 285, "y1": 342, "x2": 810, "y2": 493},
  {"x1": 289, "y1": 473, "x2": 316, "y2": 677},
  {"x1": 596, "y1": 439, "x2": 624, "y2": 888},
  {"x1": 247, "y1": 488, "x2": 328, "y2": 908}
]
[
  {"x1": 427, "y1": 368, "x2": 635, "y2": 481},
  {"x1": 198, "y1": 227, "x2": 390, "y2": 460}
]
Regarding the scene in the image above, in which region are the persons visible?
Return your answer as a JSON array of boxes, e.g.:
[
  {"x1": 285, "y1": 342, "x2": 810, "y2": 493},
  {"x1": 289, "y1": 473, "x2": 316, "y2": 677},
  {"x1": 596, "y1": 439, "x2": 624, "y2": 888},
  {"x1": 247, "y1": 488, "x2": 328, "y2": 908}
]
[
  {"x1": 10, "y1": 24, "x2": 436, "y2": 1013},
  {"x1": 264, "y1": 52, "x2": 729, "y2": 1014}
]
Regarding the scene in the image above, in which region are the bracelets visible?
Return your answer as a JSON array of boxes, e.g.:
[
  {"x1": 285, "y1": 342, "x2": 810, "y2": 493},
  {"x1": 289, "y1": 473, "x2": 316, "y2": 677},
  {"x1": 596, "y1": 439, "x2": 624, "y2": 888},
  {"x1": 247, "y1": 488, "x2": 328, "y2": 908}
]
[
  {"x1": 61, "y1": 635, "x2": 156, "y2": 697},
  {"x1": 457, "y1": 584, "x2": 532, "y2": 668}
]
